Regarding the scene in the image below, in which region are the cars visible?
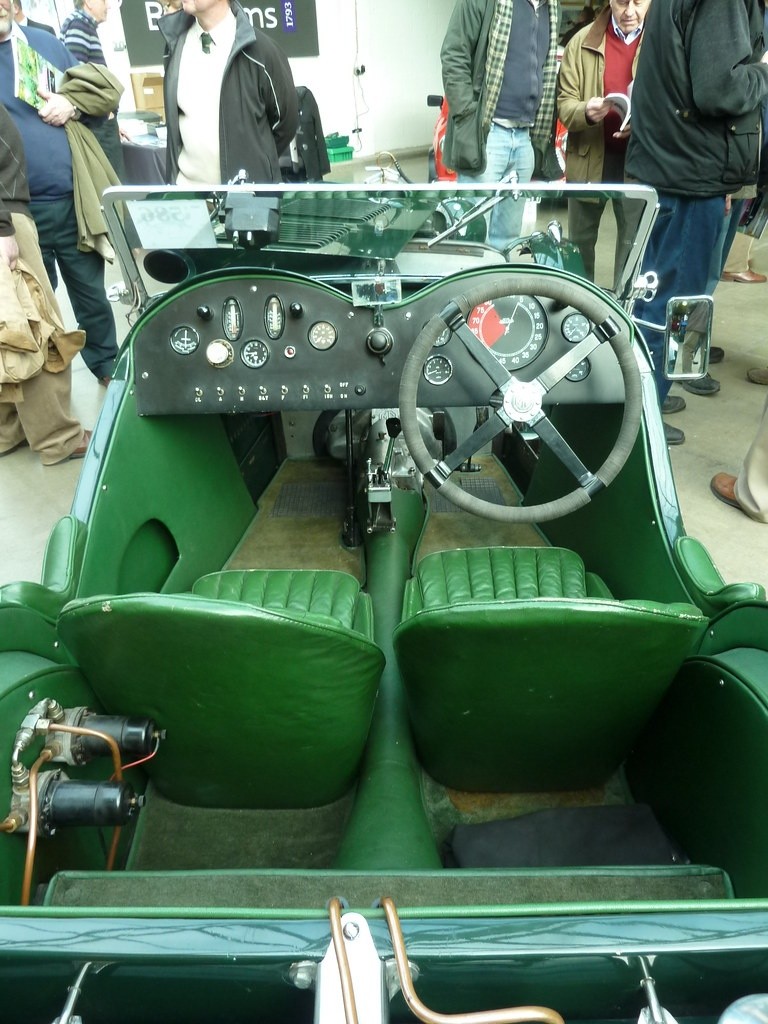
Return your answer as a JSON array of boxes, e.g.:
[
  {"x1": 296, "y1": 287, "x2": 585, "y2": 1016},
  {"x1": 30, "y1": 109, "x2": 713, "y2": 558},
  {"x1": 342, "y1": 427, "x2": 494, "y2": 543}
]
[
  {"x1": 426, "y1": 92, "x2": 569, "y2": 201},
  {"x1": 2, "y1": 149, "x2": 767, "y2": 1024}
]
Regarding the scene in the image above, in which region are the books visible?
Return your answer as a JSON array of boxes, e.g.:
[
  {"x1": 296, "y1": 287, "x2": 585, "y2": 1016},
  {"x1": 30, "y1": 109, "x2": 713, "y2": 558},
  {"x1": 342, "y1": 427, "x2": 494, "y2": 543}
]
[
  {"x1": 603, "y1": 93, "x2": 633, "y2": 132},
  {"x1": 11, "y1": 35, "x2": 66, "y2": 112}
]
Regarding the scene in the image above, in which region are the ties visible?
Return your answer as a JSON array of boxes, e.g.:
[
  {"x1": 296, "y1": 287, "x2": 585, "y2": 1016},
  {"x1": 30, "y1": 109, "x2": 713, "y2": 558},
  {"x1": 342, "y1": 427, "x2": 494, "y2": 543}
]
[{"x1": 200, "y1": 32, "x2": 213, "y2": 53}]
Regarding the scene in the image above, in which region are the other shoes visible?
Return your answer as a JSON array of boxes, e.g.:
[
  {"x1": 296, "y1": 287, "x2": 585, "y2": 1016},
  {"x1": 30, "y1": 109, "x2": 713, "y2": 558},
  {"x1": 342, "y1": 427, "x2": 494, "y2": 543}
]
[
  {"x1": 99, "y1": 376, "x2": 112, "y2": 388},
  {"x1": 747, "y1": 366, "x2": 768, "y2": 385},
  {"x1": 661, "y1": 393, "x2": 686, "y2": 414},
  {"x1": 663, "y1": 422, "x2": 685, "y2": 444},
  {"x1": 677, "y1": 375, "x2": 721, "y2": 395},
  {"x1": 708, "y1": 345, "x2": 724, "y2": 363}
]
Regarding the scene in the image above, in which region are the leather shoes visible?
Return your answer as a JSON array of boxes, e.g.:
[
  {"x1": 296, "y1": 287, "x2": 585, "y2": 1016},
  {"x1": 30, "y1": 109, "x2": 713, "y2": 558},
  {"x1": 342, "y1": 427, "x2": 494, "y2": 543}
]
[
  {"x1": 69, "y1": 428, "x2": 94, "y2": 459},
  {"x1": 721, "y1": 268, "x2": 766, "y2": 283},
  {"x1": 710, "y1": 472, "x2": 743, "y2": 510}
]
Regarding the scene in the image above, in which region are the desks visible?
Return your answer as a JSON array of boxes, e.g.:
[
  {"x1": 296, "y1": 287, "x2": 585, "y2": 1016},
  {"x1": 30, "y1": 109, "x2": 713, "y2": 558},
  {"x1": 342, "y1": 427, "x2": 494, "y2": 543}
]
[{"x1": 121, "y1": 134, "x2": 167, "y2": 185}]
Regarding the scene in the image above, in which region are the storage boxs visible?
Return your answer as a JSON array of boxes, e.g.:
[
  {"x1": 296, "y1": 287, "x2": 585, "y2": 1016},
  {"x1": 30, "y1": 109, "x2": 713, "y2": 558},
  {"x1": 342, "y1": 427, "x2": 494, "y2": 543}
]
[{"x1": 323, "y1": 132, "x2": 356, "y2": 183}]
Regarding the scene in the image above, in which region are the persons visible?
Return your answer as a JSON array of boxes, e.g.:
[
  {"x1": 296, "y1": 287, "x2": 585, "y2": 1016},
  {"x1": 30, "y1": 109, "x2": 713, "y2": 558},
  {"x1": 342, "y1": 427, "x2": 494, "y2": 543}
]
[
  {"x1": 0, "y1": 0, "x2": 127, "y2": 467},
  {"x1": 623, "y1": 1, "x2": 768, "y2": 526},
  {"x1": 155, "y1": 1, "x2": 299, "y2": 186},
  {"x1": 549, "y1": 0, "x2": 652, "y2": 315},
  {"x1": 440, "y1": 1, "x2": 562, "y2": 250}
]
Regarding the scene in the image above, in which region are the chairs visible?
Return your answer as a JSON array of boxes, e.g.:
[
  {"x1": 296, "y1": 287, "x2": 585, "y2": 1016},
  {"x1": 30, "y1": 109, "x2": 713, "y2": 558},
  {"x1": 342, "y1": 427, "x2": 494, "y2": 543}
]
[
  {"x1": 391, "y1": 545, "x2": 711, "y2": 793},
  {"x1": 58, "y1": 568, "x2": 387, "y2": 810}
]
